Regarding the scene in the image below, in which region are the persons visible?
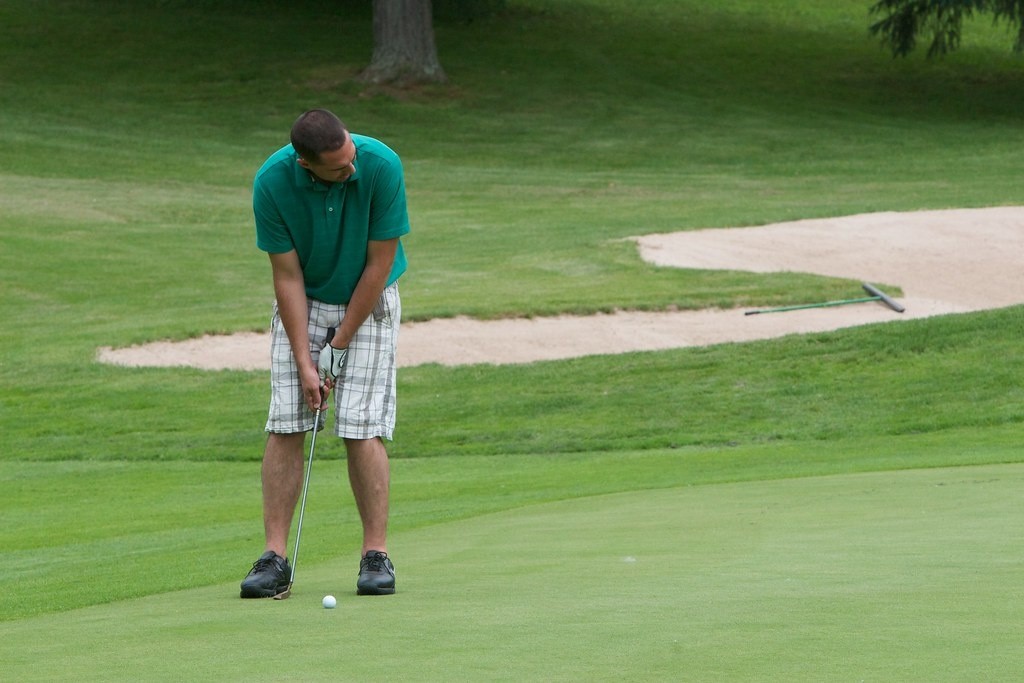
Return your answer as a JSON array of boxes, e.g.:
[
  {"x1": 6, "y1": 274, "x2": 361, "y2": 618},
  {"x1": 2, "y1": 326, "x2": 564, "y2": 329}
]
[{"x1": 241, "y1": 109, "x2": 409, "y2": 596}]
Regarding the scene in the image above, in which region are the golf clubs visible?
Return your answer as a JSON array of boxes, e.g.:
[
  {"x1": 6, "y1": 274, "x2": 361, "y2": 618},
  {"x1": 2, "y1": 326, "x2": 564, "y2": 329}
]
[{"x1": 272, "y1": 327, "x2": 336, "y2": 600}]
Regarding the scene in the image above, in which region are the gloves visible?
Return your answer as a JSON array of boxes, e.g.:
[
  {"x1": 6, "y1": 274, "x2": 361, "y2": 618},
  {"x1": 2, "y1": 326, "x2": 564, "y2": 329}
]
[{"x1": 317, "y1": 342, "x2": 348, "y2": 387}]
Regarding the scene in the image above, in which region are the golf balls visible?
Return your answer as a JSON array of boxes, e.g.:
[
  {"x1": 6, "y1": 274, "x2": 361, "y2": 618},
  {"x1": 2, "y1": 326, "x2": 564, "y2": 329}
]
[{"x1": 323, "y1": 596, "x2": 337, "y2": 608}]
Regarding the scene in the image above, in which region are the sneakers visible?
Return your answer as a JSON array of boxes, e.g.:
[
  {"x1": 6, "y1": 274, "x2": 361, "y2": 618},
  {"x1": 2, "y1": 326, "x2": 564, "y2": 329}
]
[
  {"x1": 241, "y1": 551, "x2": 292, "y2": 597},
  {"x1": 358, "y1": 548, "x2": 395, "y2": 594}
]
